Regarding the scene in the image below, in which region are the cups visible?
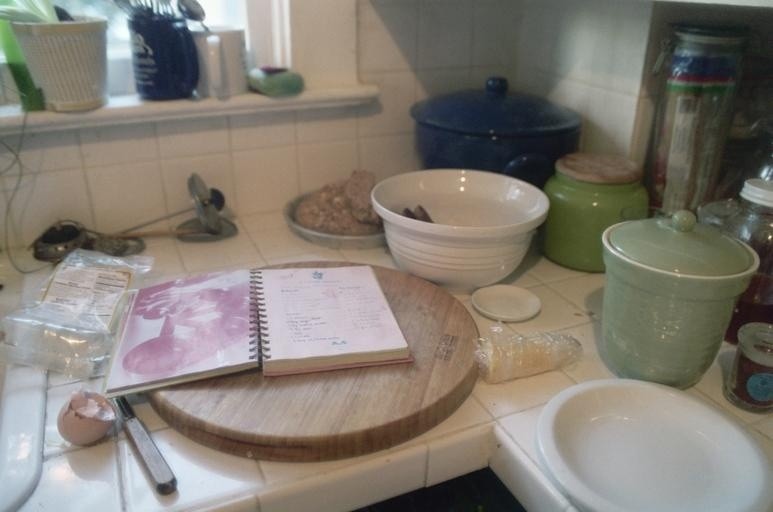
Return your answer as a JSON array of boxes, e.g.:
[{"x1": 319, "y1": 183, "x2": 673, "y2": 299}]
[
  {"x1": 601, "y1": 214, "x2": 761, "y2": 388},
  {"x1": 192, "y1": 23, "x2": 251, "y2": 100},
  {"x1": 126, "y1": 14, "x2": 199, "y2": 100}
]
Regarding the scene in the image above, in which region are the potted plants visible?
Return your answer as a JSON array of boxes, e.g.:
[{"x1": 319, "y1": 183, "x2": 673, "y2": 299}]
[{"x1": 1, "y1": 1, "x2": 109, "y2": 110}]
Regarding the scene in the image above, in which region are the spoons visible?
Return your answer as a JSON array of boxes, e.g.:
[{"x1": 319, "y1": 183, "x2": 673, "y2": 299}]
[{"x1": 177, "y1": 0, "x2": 212, "y2": 31}]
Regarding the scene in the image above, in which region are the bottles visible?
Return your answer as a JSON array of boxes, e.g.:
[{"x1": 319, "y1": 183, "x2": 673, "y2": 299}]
[
  {"x1": 643, "y1": 24, "x2": 752, "y2": 214},
  {"x1": 539, "y1": 152, "x2": 650, "y2": 272},
  {"x1": 727, "y1": 321, "x2": 773, "y2": 413},
  {"x1": 697, "y1": 177, "x2": 773, "y2": 344}
]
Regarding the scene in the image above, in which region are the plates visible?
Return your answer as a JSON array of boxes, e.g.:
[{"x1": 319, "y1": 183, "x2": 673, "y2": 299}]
[
  {"x1": 284, "y1": 182, "x2": 385, "y2": 247},
  {"x1": 538, "y1": 376, "x2": 773, "y2": 512},
  {"x1": 472, "y1": 284, "x2": 543, "y2": 323}
]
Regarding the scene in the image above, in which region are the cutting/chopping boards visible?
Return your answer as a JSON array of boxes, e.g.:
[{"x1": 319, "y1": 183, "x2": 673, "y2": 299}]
[{"x1": 148, "y1": 260, "x2": 481, "y2": 465}]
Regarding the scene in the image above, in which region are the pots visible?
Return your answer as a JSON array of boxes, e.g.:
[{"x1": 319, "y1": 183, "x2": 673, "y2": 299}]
[{"x1": 410, "y1": 74, "x2": 583, "y2": 191}]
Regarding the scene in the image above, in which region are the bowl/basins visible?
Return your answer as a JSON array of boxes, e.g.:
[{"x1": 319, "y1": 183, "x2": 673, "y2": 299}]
[{"x1": 372, "y1": 166, "x2": 550, "y2": 290}]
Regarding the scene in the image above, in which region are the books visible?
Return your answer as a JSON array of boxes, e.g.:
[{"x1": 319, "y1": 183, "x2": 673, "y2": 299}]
[{"x1": 99, "y1": 264, "x2": 414, "y2": 401}]
